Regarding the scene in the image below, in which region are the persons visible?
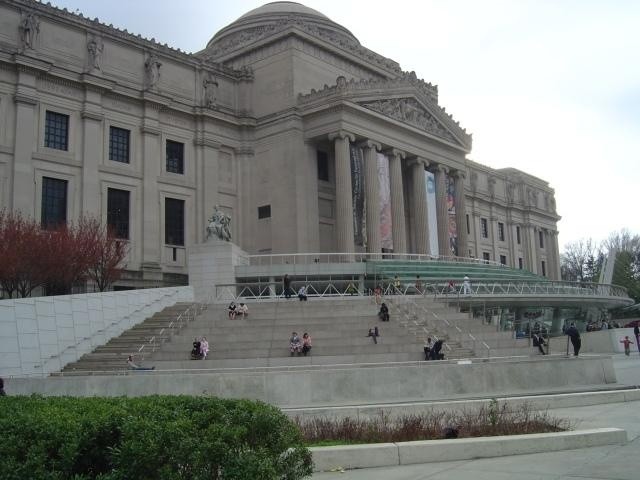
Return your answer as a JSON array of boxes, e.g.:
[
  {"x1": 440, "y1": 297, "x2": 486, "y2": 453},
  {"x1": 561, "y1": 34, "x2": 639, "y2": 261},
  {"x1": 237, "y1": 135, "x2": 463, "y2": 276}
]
[
  {"x1": 200, "y1": 337, "x2": 209, "y2": 360},
  {"x1": 462, "y1": 273, "x2": 472, "y2": 295},
  {"x1": 379, "y1": 302, "x2": 390, "y2": 322},
  {"x1": 289, "y1": 331, "x2": 301, "y2": 356},
  {"x1": 423, "y1": 337, "x2": 437, "y2": 361},
  {"x1": 191, "y1": 336, "x2": 201, "y2": 360},
  {"x1": 586, "y1": 319, "x2": 621, "y2": 333},
  {"x1": 433, "y1": 339, "x2": 444, "y2": 360},
  {"x1": 207, "y1": 204, "x2": 232, "y2": 241},
  {"x1": 374, "y1": 285, "x2": 383, "y2": 305},
  {"x1": 237, "y1": 302, "x2": 249, "y2": 321},
  {"x1": 283, "y1": 273, "x2": 293, "y2": 298},
  {"x1": 228, "y1": 301, "x2": 236, "y2": 320},
  {"x1": 391, "y1": 273, "x2": 403, "y2": 295},
  {"x1": 296, "y1": 286, "x2": 308, "y2": 302},
  {"x1": 532, "y1": 329, "x2": 548, "y2": 355},
  {"x1": 565, "y1": 322, "x2": 582, "y2": 356},
  {"x1": 414, "y1": 274, "x2": 423, "y2": 295},
  {"x1": 633, "y1": 320, "x2": 640, "y2": 354},
  {"x1": 526, "y1": 322, "x2": 548, "y2": 339},
  {"x1": 127, "y1": 356, "x2": 156, "y2": 370},
  {"x1": 301, "y1": 332, "x2": 312, "y2": 356},
  {"x1": 365, "y1": 325, "x2": 379, "y2": 345},
  {"x1": 619, "y1": 335, "x2": 634, "y2": 357}
]
